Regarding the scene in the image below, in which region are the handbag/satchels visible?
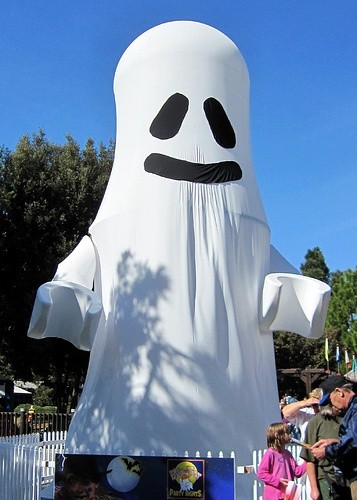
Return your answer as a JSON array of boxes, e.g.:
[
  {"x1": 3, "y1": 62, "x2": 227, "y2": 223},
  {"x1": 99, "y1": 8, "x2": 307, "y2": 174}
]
[
  {"x1": 285, "y1": 481, "x2": 302, "y2": 500},
  {"x1": 328, "y1": 480, "x2": 353, "y2": 500}
]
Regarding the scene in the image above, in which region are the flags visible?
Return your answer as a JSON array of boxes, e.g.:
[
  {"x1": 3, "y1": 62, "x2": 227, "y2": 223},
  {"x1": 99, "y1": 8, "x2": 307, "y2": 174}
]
[
  {"x1": 324, "y1": 337, "x2": 329, "y2": 361},
  {"x1": 352, "y1": 356, "x2": 357, "y2": 371},
  {"x1": 336, "y1": 346, "x2": 340, "y2": 364},
  {"x1": 345, "y1": 352, "x2": 350, "y2": 368}
]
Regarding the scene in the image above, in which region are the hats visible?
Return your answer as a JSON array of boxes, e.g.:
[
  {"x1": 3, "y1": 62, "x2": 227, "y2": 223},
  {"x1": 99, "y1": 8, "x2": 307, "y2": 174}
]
[{"x1": 317, "y1": 376, "x2": 347, "y2": 407}]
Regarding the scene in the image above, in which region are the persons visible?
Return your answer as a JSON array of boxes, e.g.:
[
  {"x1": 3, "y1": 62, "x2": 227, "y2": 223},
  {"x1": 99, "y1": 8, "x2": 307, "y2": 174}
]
[
  {"x1": 279, "y1": 389, "x2": 299, "y2": 412},
  {"x1": 299, "y1": 401, "x2": 351, "y2": 500},
  {"x1": 282, "y1": 386, "x2": 324, "y2": 500},
  {"x1": 258, "y1": 421, "x2": 307, "y2": 500},
  {"x1": 310, "y1": 375, "x2": 357, "y2": 500}
]
